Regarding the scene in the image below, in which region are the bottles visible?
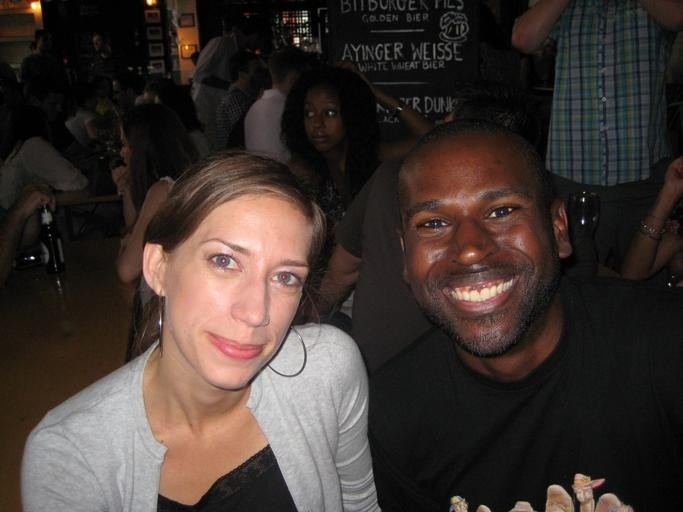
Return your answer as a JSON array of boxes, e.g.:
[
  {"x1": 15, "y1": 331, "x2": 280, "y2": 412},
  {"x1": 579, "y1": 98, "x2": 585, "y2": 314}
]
[{"x1": 39, "y1": 203, "x2": 66, "y2": 275}]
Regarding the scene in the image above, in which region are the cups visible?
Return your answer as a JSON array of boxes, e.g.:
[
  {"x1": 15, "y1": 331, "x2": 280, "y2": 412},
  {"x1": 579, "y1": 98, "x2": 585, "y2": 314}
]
[{"x1": 566, "y1": 189, "x2": 602, "y2": 239}]
